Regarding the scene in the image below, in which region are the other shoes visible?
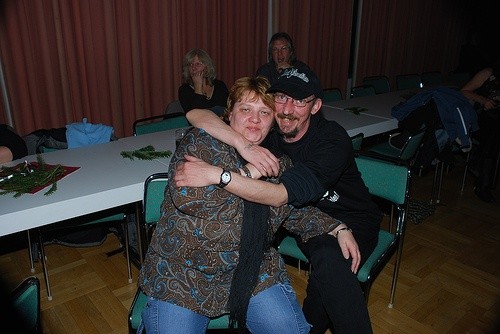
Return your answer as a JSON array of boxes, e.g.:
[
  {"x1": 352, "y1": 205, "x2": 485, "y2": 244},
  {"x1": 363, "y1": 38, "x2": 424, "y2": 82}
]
[{"x1": 474, "y1": 185, "x2": 495, "y2": 204}]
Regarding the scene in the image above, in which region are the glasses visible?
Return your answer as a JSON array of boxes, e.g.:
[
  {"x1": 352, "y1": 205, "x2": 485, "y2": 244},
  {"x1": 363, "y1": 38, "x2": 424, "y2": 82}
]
[
  {"x1": 272, "y1": 45, "x2": 289, "y2": 53},
  {"x1": 187, "y1": 63, "x2": 203, "y2": 68},
  {"x1": 273, "y1": 95, "x2": 317, "y2": 107}
]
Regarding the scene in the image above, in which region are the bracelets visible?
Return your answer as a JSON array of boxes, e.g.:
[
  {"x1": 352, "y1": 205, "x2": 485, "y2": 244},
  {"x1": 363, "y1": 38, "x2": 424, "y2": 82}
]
[
  {"x1": 335, "y1": 227, "x2": 352, "y2": 237},
  {"x1": 240, "y1": 165, "x2": 252, "y2": 177}
]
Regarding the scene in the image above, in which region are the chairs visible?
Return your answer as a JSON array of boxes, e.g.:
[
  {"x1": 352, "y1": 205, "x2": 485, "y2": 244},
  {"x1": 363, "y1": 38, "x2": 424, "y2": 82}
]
[
  {"x1": 275, "y1": 71, "x2": 484, "y2": 310},
  {"x1": 0, "y1": 110, "x2": 187, "y2": 334},
  {"x1": 127, "y1": 172, "x2": 249, "y2": 334}
]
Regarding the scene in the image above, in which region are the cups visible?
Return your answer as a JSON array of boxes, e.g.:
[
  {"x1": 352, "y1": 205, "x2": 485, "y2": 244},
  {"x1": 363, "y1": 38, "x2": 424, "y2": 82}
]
[{"x1": 176, "y1": 129, "x2": 186, "y2": 149}]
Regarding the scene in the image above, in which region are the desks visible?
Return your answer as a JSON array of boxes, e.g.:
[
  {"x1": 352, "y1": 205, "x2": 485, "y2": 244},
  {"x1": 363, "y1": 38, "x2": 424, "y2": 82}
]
[
  {"x1": 322, "y1": 80, "x2": 476, "y2": 148},
  {"x1": 118, "y1": 106, "x2": 396, "y2": 168},
  {"x1": 0, "y1": 140, "x2": 169, "y2": 237}
]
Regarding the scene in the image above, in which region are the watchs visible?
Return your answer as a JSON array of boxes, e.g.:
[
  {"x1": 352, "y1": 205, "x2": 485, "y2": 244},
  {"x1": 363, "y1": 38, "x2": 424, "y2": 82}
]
[{"x1": 216, "y1": 168, "x2": 231, "y2": 188}]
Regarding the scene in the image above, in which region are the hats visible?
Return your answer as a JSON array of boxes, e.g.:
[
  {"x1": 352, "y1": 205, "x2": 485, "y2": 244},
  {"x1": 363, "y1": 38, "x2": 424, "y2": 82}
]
[{"x1": 264, "y1": 67, "x2": 321, "y2": 100}]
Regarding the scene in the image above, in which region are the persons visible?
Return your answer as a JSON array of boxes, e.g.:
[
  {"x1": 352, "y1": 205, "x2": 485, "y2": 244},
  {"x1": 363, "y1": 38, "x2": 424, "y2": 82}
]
[
  {"x1": 0, "y1": 125, "x2": 27, "y2": 165},
  {"x1": 458, "y1": 54, "x2": 500, "y2": 203},
  {"x1": 173, "y1": 69, "x2": 381, "y2": 334},
  {"x1": 256, "y1": 32, "x2": 311, "y2": 85},
  {"x1": 136, "y1": 80, "x2": 361, "y2": 334},
  {"x1": 177, "y1": 47, "x2": 229, "y2": 127}
]
